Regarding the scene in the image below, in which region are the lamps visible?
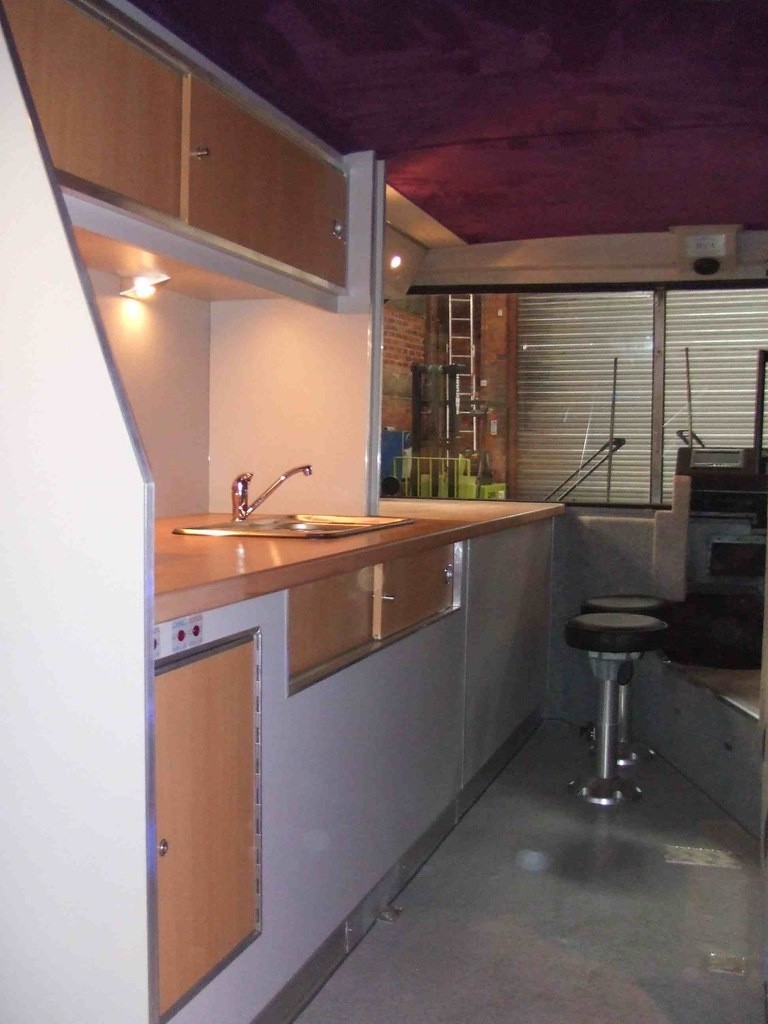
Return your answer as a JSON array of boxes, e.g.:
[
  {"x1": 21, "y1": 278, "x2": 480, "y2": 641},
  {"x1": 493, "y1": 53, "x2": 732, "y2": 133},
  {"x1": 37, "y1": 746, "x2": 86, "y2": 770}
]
[{"x1": 118, "y1": 274, "x2": 172, "y2": 302}]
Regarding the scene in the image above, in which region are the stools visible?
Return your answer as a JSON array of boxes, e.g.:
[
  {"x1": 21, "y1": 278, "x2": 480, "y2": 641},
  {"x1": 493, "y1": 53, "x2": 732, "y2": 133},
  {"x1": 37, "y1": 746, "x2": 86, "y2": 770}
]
[
  {"x1": 564, "y1": 612, "x2": 671, "y2": 805},
  {"x1": 578, "y1": 594, "x2": 673, "y2": 766}
]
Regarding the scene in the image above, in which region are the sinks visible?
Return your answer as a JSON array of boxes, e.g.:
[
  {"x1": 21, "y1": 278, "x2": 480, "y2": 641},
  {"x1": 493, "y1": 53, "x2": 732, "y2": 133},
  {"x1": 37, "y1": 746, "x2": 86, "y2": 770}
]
[
  {"x1": 253, "y1": 512, "x2": 415, "y2": 526},
  {"x1": 171, "y1": 520, "x2": 377, "y2": 539}
]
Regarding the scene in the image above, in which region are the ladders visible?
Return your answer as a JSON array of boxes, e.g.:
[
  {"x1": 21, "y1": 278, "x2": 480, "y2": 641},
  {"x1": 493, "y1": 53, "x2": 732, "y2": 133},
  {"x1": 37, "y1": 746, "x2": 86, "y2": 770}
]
[{"x1": 445, "y1": 294, "x2": 478, "y2": 478}]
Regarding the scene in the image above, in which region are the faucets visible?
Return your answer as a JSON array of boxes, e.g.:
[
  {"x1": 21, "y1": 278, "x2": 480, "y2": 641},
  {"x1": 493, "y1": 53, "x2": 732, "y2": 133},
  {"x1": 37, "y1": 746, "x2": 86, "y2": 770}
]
[{"x1": 232, "y1": 464, "x2": 312, "y2": 522}]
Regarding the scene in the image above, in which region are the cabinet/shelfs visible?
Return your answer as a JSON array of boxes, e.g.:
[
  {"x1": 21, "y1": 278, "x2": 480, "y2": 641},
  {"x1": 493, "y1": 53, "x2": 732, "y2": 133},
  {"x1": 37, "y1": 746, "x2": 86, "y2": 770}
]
[
  {"x1": 0, "y1": 1, "x2": 351, "y2": 293},
  {"x1": 288, "y1": 541, "x2": 453, "y2": 680}
]
